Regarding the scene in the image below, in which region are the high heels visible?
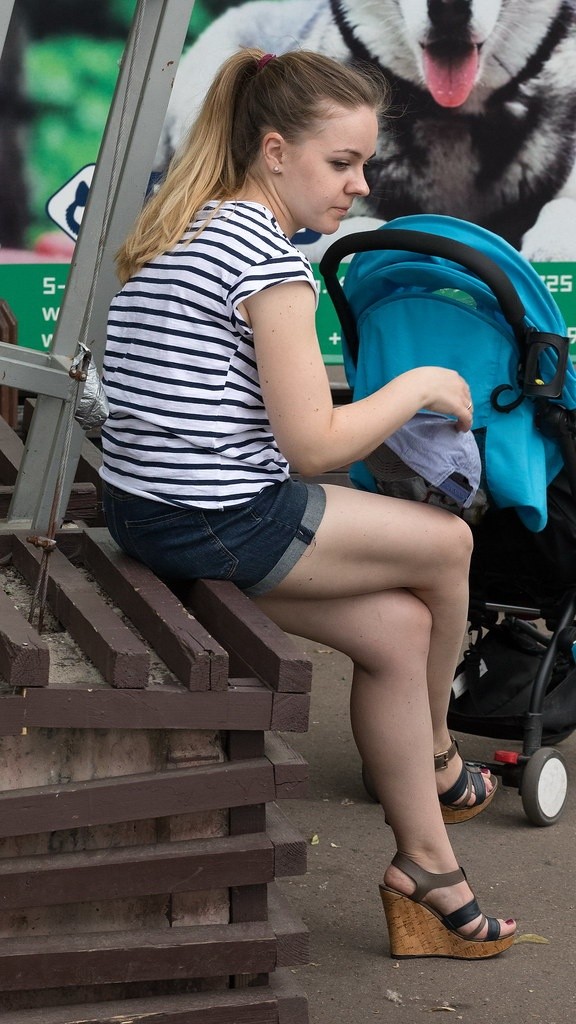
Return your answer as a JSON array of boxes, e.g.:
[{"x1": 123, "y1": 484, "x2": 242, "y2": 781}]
[
  {"x1": 378, "y1": 852, "x2": 516, "y2": 958},
  {"x1": 383, "y1": 734, "x2": 498, "y2": 826}
]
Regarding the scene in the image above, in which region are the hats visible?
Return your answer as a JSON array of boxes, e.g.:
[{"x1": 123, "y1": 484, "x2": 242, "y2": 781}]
[{"x1": 372, "y1": 412, "x2": 483, "y2": 509}]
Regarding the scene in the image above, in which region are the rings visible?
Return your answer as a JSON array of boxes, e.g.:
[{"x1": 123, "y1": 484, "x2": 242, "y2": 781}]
[{"x1": 467, "y1": 403, "x2": 472, "y2": 409}]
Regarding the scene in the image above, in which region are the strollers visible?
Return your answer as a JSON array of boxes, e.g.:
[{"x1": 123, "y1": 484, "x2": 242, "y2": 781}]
[{"x1": 319, "y1": 209, "x2": 576, "y2": 831}]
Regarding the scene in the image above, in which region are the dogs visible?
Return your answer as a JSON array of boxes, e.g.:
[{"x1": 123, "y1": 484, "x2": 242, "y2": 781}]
[{"x1": 150, "y1": 0, "x2": 576, "y2": 253}]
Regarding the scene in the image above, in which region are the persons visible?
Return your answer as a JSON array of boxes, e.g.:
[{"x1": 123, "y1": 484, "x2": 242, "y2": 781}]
[{"x1": 98, "y1": 48, "x2": 519, "y2": 959}]
